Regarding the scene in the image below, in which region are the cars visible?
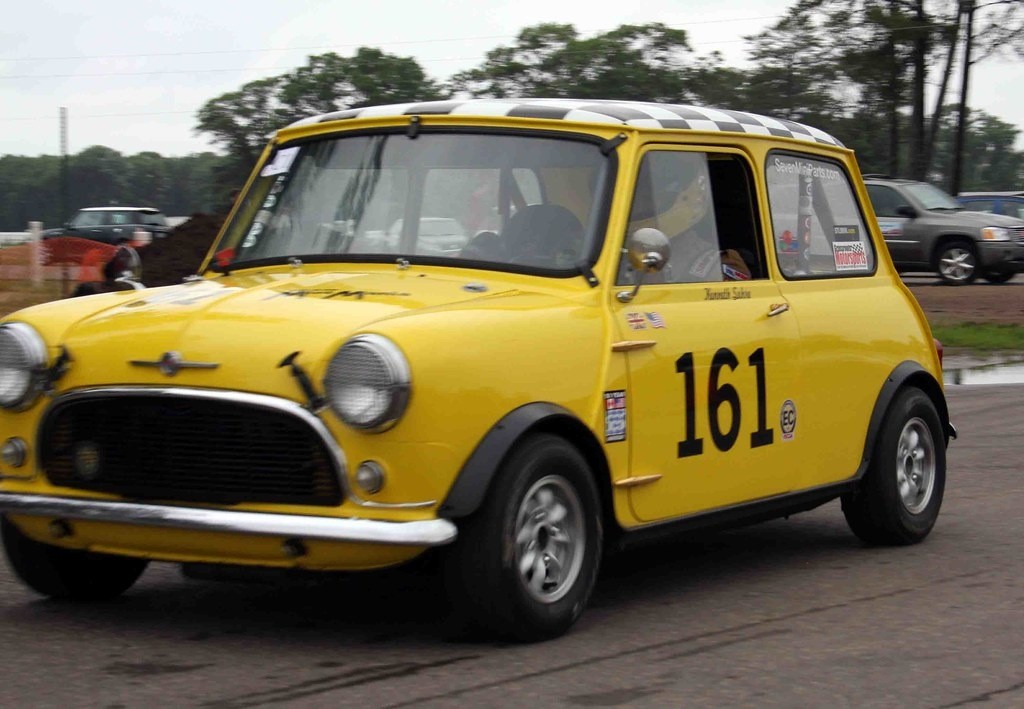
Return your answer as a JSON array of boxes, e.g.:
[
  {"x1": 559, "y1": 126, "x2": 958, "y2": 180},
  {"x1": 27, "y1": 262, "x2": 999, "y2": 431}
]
[
  {"x1": 0, "y1": 97, "x2": 953, "y2": 649},
  {"x1": 955, "y1": 196, "x2": 1023, "y2": 219},
  {"x1": 387, "y1": 217, "x2": 473, "y2": 257}
]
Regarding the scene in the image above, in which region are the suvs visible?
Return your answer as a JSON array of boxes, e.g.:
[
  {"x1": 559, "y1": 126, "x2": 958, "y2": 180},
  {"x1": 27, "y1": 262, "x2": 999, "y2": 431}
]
[
  {"x1": 775, "y1": 173, "x2": 1024, "y2": 286},
  {"x1": 40, "y1": 208, "x2": 173, "y2": 267}
]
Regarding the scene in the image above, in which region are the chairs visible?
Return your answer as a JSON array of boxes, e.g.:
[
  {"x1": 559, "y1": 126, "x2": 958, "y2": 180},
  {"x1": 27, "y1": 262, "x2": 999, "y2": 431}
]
[{"x1": 502, "y1": 203, "x2": 586, "y2": 267}]
